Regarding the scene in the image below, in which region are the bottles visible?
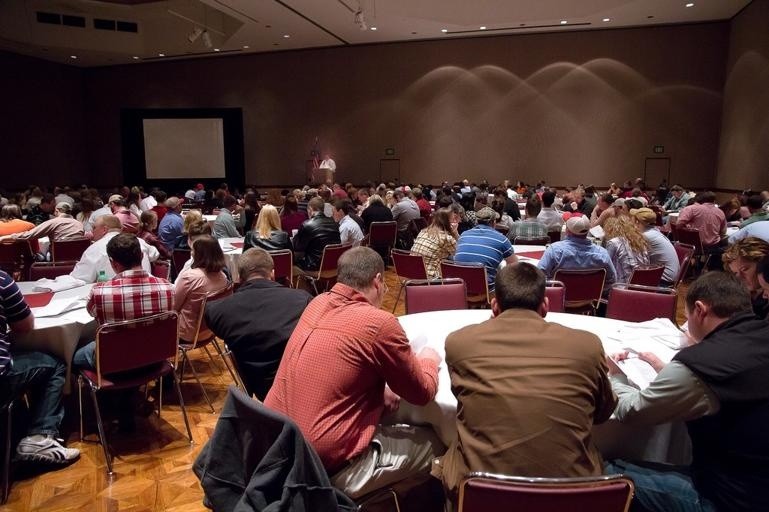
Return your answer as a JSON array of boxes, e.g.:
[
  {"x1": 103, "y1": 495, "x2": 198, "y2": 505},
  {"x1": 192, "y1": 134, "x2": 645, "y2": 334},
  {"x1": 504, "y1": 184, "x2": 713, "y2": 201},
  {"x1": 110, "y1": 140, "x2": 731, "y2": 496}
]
[{"x1": 98, "y1": 271, "x2": 108, "y2": 282}]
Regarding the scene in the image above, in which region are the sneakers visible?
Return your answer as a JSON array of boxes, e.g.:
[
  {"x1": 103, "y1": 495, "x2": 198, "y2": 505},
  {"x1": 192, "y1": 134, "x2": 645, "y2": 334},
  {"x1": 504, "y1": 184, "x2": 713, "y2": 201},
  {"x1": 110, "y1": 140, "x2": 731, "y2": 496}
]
[{"x1": 14, "y1": 434, "x2": 81, "y2": 465}]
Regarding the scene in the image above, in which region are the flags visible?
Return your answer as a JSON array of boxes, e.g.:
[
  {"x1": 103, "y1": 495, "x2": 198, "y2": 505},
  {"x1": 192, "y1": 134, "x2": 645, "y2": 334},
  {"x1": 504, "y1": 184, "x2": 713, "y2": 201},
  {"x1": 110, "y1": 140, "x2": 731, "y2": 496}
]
[{"x1": 310, "y1": 136, "x2": 320, "y2": 185}]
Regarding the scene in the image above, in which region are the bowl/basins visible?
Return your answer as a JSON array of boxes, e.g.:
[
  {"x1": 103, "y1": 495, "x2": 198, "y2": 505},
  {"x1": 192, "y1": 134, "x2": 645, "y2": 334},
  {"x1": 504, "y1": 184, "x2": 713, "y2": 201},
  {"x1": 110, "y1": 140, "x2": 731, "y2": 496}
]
[{"x1": 20, "y1": 286, "x2": 52, "y2": 306}]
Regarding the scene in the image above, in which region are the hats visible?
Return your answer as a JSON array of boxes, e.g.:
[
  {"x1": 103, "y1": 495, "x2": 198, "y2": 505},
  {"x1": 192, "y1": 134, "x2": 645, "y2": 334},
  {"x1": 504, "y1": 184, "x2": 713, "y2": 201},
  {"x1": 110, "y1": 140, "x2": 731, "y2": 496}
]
[
  {"x1": 475, "y1": 206, "x2": 500, "y2": 222},
  {"x1": 629, "y1": 207, "x2": 657, "y2": 225},
  {"x1": 54, "y1": 201, "x2": 72, "y2": 212},
  {"x1": 107, "y1": 194, "x2": 125, "y2": 208},
  {"x1": 164, "y1": 196, "x2": 185, "y2": 210},
  {"x1": 562, "y1": 211, "x2": 590, "y2": 235}
]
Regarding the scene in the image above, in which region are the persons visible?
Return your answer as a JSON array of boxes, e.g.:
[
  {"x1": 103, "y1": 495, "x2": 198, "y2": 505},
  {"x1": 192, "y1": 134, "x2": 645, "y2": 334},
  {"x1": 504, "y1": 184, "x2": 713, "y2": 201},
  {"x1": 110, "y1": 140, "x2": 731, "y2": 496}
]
[
  {"x1": 602, "y1": 271, "x2": 767, "y2": 511},
  {"x1": 152, "y1": 237, "x2": 233, "y2": 396},
  {"x1": 0, "y1": 270, "x2": 81, "y2": 466},
  {"x1": 429, "y1": 262, "x2": 619, "y2": 512},
  {"x1": 1, "y1": 183, "x2": 769, "y2": 345},
  {"x1": 202, "y1": 247, "x2": 314, "y2": 401},
  {"x1": 261, "y1": 246, "x2": 442, "y2": 509},
  {"x1": 70, "y1": 233, "x2": 174, "y2": 443},
  {"x1": 319, "y1": 153, "x2": 336, "y2": 184}
]
